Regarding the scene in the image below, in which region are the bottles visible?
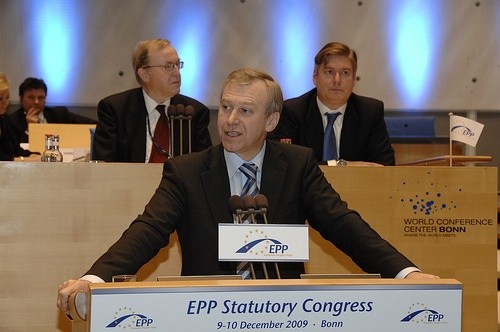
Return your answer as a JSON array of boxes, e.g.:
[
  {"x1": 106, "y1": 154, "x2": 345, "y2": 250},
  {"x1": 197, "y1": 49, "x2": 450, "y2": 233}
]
[
  {"x1": 50, "y1": 135, "x2": 63, "y2": 162},
  {"x1": 41, "y1": 134, "x2": 53, "y2": 162}
]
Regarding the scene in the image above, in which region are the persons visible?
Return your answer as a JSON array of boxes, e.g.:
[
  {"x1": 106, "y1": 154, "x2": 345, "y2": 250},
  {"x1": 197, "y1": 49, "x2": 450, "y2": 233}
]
[
  {"x1": 272, "y1": 42, "x2": 397, "y2": 168},
  {"x1": 57, "y1": 67, "x2": 440, "y2": 315},
  {"x1": 0, "y1": 72, "x2": 39, "y2": 161},
  {"x1": 92, "y1": 38, "x2": 214, "y2": 162},
  {"x1": 9, "y1": 77, "x2": 99, "y2": 144}
]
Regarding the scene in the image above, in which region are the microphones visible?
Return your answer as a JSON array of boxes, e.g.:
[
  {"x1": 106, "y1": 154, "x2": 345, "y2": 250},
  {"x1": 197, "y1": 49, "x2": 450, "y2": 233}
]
[
  {"x1": 167, "y1": 104, "x2": 194, "y2": 158},
  {"x1": 228, "y1": 194, "x2": 280, "y2": 280}
]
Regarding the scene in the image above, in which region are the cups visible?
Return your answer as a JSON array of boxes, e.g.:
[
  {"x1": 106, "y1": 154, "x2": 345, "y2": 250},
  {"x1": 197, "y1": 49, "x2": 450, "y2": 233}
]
[{"x1": 112, "y1": 275, "x2": 138, "y2": 282}]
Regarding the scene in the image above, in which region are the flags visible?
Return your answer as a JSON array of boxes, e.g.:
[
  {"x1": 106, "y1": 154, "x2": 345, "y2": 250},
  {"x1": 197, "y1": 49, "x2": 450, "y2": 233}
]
[{"x1": 449, "y1": 114, "x2": 484, "y2": 147}]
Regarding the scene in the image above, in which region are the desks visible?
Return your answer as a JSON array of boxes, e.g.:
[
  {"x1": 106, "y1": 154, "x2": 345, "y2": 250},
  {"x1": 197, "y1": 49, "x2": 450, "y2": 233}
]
[{"x1": 0, "y1": 161, "x2": 498, "y2": 332}]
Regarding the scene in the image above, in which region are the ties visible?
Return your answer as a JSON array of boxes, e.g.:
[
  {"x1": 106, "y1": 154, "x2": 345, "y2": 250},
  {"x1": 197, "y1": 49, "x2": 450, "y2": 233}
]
[
  {"x1": 322, "y1": 112, "x2": 342, "y2": 164},
  {"x1": 236, "y1": 163, "x2": 259, "y2": 280},
  {"x1": 148, "y1": 105, "x2": 170, "y2": 163}
]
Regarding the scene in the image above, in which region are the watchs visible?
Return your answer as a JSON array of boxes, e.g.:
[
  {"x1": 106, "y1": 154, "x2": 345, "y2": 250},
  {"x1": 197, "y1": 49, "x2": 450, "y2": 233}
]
[{"x1": 336, "y1": 158, "x2": 348, "y2": 166}]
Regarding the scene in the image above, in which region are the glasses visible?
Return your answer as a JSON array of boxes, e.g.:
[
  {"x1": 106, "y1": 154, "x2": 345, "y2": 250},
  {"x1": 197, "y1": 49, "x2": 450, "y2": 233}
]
[{"x1": 142, "y1": 62, "x2": 184, "y2": 72}]
